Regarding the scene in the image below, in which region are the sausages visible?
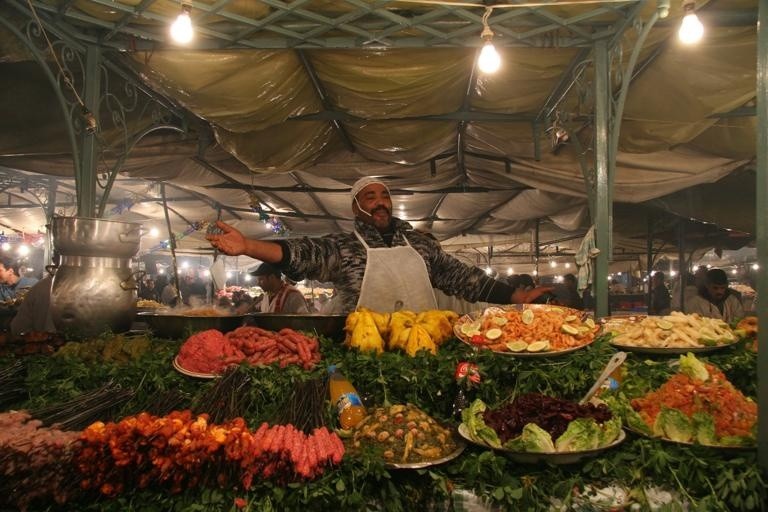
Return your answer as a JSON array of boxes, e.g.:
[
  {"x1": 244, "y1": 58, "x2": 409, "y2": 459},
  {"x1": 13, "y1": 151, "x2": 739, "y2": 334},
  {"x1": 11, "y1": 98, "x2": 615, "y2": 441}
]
[
  {"x1": 241, "y1": 422, "x2": 345, "y2": 489},
  {"x1": 222, "y1": 327, "x2": 321, "y2": 373}
]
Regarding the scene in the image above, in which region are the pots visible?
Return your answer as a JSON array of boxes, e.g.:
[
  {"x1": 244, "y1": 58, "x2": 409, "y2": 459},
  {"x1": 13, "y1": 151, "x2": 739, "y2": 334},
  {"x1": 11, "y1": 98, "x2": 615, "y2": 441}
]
[
  {"x1": 43, "y1": 253, "x2": 147, "y2": 339},
  {"x1": 50, "y1": 213, "x2": 151, "y2": 258}
]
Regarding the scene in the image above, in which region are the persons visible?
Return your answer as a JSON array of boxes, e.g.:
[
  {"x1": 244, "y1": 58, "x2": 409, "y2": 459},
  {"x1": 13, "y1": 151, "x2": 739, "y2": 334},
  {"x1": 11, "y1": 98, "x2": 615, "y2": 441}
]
[
  {"x1": 669, "y1": 273, "x2": 698, "y2": 310},
  {"x1": 201, "y1": 173, "x2": 558, "y2": 318},
  {"x1": 0, "y1": 256, "x2": 39, "y2": 304},
  {"x1": 503, "y1": 269, "x2": 591, "y2": 310},
  {"x1": 137, "y1": 262, "x2": 331, "y2": 317},
  {"x1": 647, "y1": 271, "x2": 671, "y2": 318},
  {"x1": 689, "y1": 265, "x2": 711, "y2": 288},
  {"x1": 691, "y1": 269, "x2": 745, "y2": 324}
]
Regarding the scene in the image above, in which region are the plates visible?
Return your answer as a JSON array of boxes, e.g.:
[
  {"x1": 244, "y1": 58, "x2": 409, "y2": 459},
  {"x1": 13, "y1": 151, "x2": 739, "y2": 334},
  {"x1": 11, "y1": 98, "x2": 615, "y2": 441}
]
[
  {"x1": 173, "y1": 351, "x2": 232, "y2": 379},
  {"x1": 595, "y1": 314, "x2": 741, "y2": 354},
  {"x1": 457, "y1": 416, "x2": 626, "y2": 464},
  {"x1": 342, "y1": 425, "x2": 468, "y2": 469},
  {"x1": 454, "y1": 302, "x2": 604, "y2": 359},
  {"x1": 619, "y1": 396, "x2": 760, "y2": 449}
]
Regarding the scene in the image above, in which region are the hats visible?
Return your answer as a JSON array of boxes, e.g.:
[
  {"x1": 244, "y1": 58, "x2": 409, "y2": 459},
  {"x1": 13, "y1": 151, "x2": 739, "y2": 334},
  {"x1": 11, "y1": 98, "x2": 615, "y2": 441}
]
[
  {"x1": 704, "y1": 269, "x2": 728, "y2": 285},
  {"x1": 349, "y1": 176, "x2": 390, "y2": 207},
  {"x1": 250, "y1": 262, "x2": 282, "y2": 275}
]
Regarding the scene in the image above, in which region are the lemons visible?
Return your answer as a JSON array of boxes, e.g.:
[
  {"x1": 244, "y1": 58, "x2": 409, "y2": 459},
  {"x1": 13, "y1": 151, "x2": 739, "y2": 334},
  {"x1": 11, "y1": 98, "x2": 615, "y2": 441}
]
[
  {"x1": 656, "y1": 320, "x2": 673, "y2": 330},
  {"x1": 461, "y1": 307, "x2": 596, "y2": 353}
]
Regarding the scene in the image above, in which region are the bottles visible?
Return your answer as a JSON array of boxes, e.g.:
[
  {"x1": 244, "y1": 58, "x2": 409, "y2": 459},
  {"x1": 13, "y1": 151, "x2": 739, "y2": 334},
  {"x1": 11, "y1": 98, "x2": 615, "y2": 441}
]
[{"x1": 327, "y1": 364, "x2": 367, "y2": 432}]
